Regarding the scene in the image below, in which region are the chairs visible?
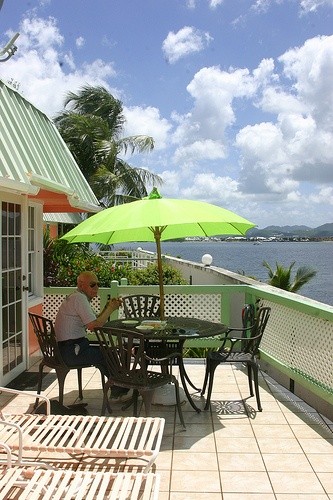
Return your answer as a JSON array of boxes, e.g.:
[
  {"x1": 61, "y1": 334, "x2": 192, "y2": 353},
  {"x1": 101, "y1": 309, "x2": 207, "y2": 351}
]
[
  {"x1": 28, "y1": 311, "x2": 113, "y2": 413},
  {"x1": 118, "y1": 294, "x2": 164, "y2": 319},
  {"x1": 91, "y1": 327, "x2": 187, "y2": 430},
  {"x1": 200, "y1": 307, "x2": 271, "y2": 411}
]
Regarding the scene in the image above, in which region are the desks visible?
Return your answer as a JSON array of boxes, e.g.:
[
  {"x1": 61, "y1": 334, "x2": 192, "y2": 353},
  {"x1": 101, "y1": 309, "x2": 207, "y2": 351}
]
[{"x1": 99, "y1": 317, "x2": 229, "y2": 413}]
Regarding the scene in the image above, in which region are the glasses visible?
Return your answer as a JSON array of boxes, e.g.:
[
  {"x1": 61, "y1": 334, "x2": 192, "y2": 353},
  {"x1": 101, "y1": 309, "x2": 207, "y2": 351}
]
[{"x1": 85, "y1": 280, "x2": 100, "y2": 288}]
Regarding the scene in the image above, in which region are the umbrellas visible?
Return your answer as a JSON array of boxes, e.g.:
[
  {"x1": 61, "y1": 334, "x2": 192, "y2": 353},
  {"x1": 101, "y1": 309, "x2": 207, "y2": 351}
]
[{"x1": 59, "y1": 189, "x2": 259, "y2": 384}]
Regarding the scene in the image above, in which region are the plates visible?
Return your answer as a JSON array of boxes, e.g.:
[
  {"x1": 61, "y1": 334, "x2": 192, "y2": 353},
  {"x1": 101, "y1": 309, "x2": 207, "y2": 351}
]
[
  {"x1": 122, "y1": 320, "x2": 138, "y2": 324},
  {"x1": 136, "y1": 326, "x2": 154, "y2": 330}
]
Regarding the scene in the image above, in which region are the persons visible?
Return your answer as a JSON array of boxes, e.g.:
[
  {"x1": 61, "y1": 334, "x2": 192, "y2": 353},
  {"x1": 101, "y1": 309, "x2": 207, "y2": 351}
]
[{"x1": 55, "y1": 271, "x2": 130, "y2": 399}]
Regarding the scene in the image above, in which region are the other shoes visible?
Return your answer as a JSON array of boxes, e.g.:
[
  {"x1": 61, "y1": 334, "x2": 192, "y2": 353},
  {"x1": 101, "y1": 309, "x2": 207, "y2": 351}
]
[{"x1": 110, "y1": 385, "x2": 130, "y2": 398}]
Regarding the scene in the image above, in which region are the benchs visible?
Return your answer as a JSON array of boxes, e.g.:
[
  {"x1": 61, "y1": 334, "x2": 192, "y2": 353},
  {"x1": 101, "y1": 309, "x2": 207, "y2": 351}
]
[{"x1": 0, "y1": 387, "x2": 165, "y2": 500}]
